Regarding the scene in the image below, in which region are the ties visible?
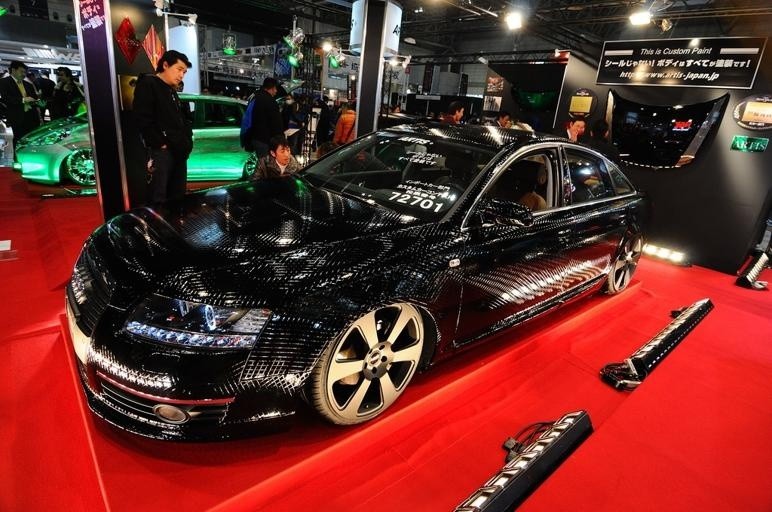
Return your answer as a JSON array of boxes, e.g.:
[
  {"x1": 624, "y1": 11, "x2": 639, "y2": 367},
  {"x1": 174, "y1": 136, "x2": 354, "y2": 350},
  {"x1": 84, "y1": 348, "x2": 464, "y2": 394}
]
[{"x1": 18, "y1": 81, "x2": 29, "y2": 112}]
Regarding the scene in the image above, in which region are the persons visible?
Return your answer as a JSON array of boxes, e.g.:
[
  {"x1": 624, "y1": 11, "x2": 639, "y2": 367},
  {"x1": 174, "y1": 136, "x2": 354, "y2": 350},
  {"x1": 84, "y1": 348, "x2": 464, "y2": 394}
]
[
  {"x1": 553, "y1": 115, "x2": 588, "y2": 143},
  {"x1": 131, "y1": 49, "x2": 195, "y2": 204},
  {"x1": 201, "y1": 77, "x2": 486, "y2": 150},
  {"x1": 489, "y1": 111, "x2": 511, "y2": 128},
  {"x1": 0, "y1": 58, "x2": 86, "y2": 166},
  {"x1": 581, "y1": 118, "x2": 619, "y2": 169},
  {"x1": 250, "y1": 136, "x2": 303, "y2": 180}
]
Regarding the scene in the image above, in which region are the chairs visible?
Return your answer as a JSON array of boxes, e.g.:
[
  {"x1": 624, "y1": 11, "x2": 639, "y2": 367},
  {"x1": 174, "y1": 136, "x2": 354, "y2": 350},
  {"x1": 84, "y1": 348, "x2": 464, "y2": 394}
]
[
  {"x1": 506, "y1": 158, "x2": 547, "y2": 213},
  {"x1": 437, "y1": 151, "x2": 474, "y2": 192}
]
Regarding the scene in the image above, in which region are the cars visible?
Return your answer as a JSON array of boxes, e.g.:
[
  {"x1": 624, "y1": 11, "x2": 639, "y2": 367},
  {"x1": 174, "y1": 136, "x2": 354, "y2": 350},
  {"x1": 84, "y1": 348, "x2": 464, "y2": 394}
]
[
  {"x1": 64, "y1": 118, "x2": 646, "y2": 446},
  {"x1": 14, "y1": 93, "x2": 258, "y2": 186}
]
